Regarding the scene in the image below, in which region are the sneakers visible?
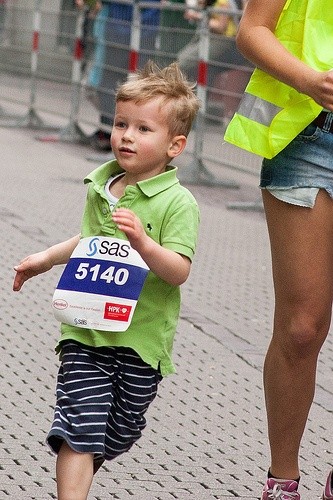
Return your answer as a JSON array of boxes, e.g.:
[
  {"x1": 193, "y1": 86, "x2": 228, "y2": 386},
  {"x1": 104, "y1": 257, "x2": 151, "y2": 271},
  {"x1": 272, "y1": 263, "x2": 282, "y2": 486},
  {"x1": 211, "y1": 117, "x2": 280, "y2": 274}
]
[
  {"x1": 262, "y1": 478, "x2": 300, "y2": 500},
  {"x1": 321, "y1": 469, "x2": 333, "y2": 500}
]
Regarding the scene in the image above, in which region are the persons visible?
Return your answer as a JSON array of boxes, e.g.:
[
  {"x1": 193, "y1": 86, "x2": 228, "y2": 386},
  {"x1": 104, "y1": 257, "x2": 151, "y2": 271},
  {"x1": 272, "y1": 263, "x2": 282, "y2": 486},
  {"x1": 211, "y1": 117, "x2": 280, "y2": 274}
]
[
  {"x1": 77, "y1": 0, "x2": 255, "y2": 155},
  {"x1": 220, "y1": 0, "x2": 333, "y2": 500},
  {"x1": 12, "y1": 59, "x2": 200, "y2": 500}
]
